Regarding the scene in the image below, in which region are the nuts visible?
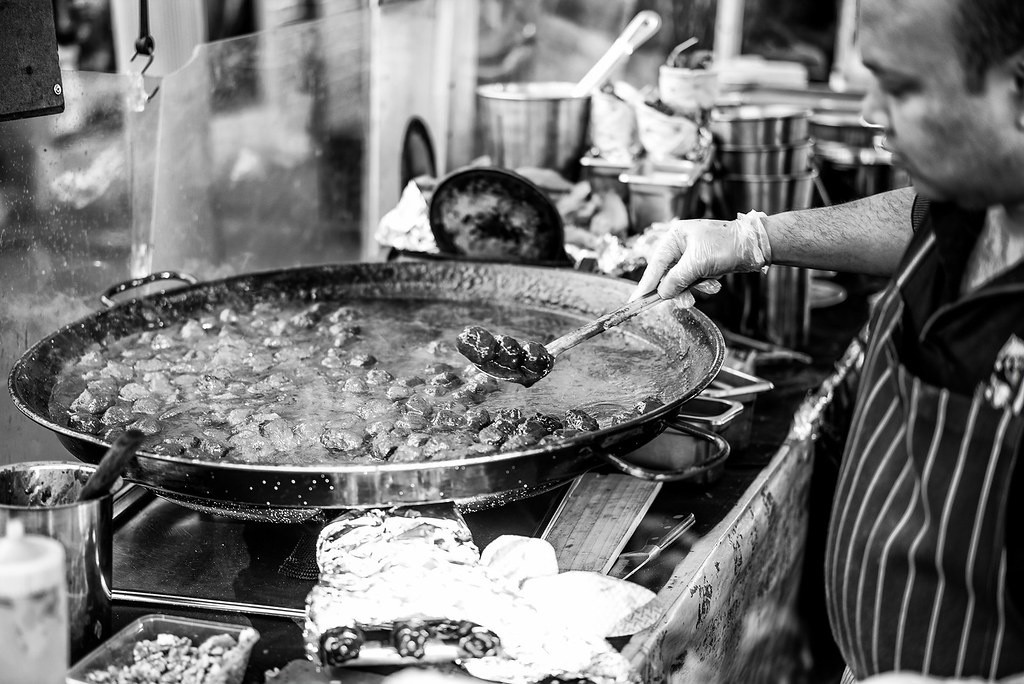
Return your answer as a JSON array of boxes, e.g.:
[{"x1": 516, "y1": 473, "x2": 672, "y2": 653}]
[{"x1": 84, "y1": 632, "x2": 242, "y2": 684}]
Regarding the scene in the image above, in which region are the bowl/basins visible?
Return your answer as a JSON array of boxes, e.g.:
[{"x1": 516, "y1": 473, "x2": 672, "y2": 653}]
[{"x1": 69, "y1": 613, "x2": 260, "y2": 684}]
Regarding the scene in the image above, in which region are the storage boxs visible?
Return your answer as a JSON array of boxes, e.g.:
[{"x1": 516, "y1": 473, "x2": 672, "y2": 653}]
[{"x1": 64, "y1": 611, "x2": 262, "y2": 684}]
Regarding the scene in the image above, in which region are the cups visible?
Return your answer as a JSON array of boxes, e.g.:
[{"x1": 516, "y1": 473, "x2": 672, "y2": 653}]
[{"x1": 0, "y1": 460, "x2": 123, "y2": 667}]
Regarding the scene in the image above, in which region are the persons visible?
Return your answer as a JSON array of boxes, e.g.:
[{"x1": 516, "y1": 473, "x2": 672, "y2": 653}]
[{"x1": 628, "y1": 0, "x2": 1024, "y2": 684}]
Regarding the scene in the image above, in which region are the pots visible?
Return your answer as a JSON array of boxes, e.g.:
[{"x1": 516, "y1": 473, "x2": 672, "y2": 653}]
[
  {"x1": 473, "y1": 67, "x2": 910, "y2": 348},
  {"x1": 9, "y1": 261, "x2": 734, "y2": 511}
]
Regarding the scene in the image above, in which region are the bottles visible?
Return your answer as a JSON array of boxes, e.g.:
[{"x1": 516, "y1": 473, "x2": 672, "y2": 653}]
[{"x1": 0, "y1": 521, "x2": 69, "y2": 684}]
